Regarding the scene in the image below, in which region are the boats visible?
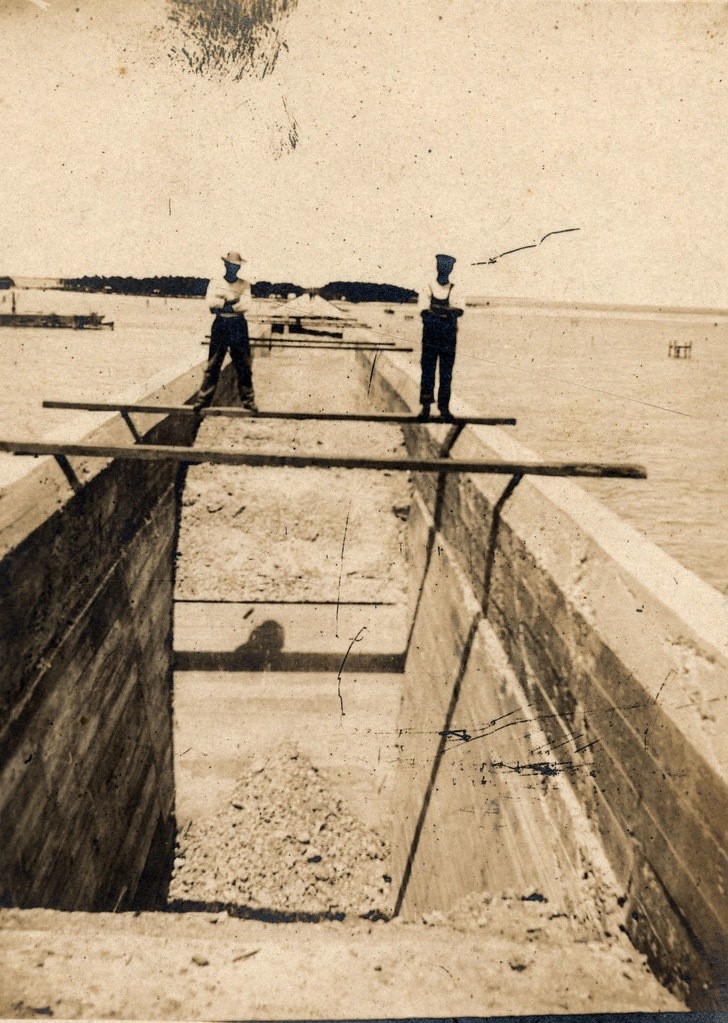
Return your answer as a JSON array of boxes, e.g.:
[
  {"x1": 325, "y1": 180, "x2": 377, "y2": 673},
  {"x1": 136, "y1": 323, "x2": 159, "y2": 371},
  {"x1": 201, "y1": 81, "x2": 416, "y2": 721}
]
[
  {"x1": 384, "y1": 309, "x2": 394, "y2": 314},
  {"x1": 0, "y1": 276, "x2": 113, "y2": 330}
]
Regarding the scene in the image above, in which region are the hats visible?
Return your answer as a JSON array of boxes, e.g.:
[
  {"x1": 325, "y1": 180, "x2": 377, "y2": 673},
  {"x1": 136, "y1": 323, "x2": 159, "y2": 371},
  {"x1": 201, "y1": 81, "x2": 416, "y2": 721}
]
[
  {"x1": 221, "y1": 252, "x2": 247, "y2": 265},
  {"x1": 436, "y1": 254, "x2": 456, "y2": 263}
]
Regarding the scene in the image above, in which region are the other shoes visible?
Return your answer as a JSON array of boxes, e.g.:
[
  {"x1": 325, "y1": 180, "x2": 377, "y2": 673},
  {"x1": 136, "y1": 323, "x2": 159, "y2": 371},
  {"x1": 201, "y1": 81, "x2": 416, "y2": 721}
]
[
  {"x1": 441, "y1": 412, "x2": 458, "y2": 425},
  {"x1": 418, "y1": 411, "x2": 430, "y2": 418},
  {"x1": 244, "y1": 403, "x2": 258, "y2": 411},
  {"x1": 193, "y1": 402, "x2": 210, "y2": 411}
]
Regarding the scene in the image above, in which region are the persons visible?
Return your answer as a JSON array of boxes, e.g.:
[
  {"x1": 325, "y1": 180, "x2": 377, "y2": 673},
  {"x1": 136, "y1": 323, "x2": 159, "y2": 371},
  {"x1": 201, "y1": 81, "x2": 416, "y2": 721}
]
[
  {"x1": 194, "y1": 250, "x2": 261, "y2": 413},
  {"x1": 416, "y1": 252, "x2": 465, "y2": 425}
]
